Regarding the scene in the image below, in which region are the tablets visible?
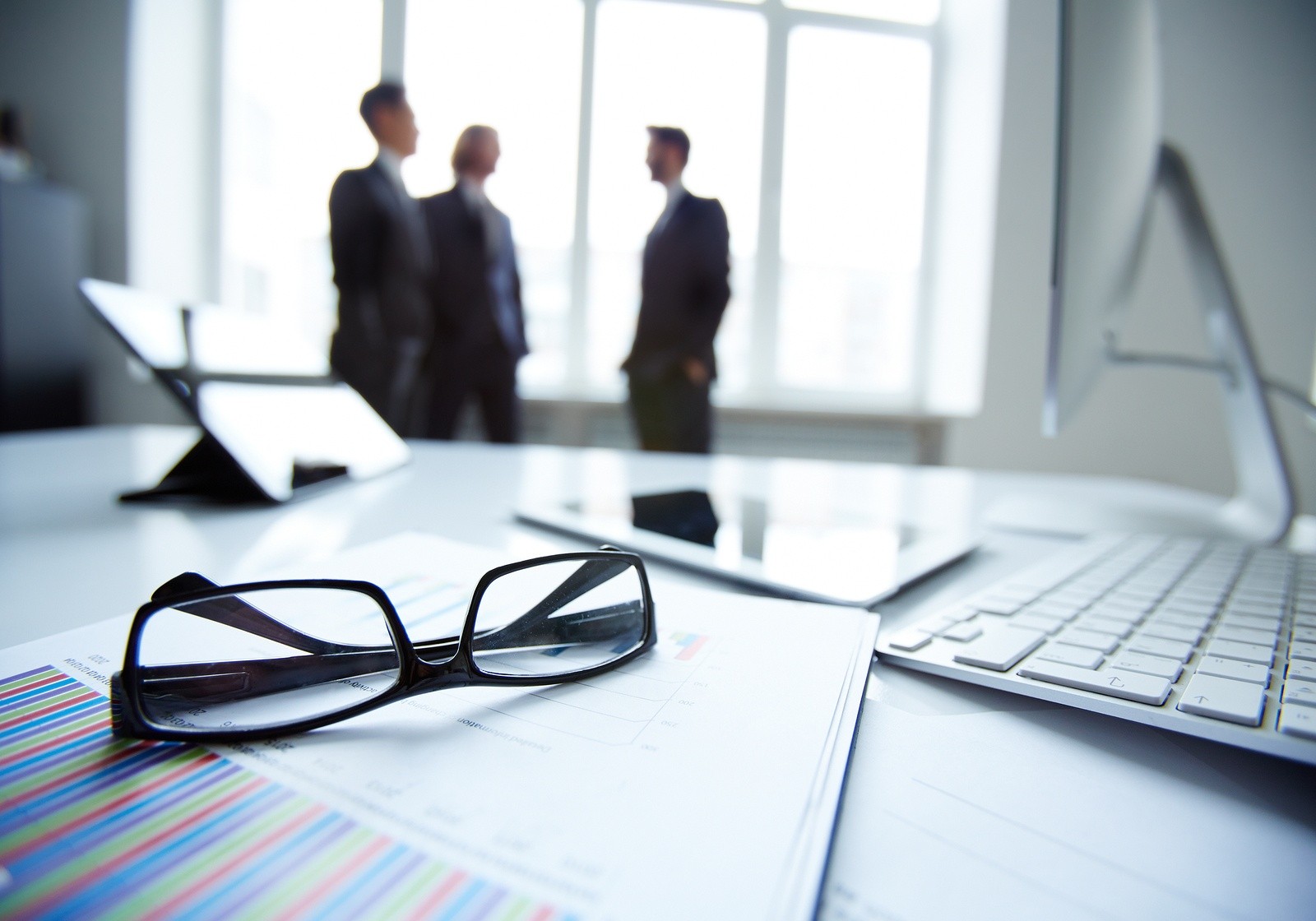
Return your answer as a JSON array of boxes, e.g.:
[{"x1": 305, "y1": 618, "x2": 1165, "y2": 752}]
[
  {"x1": 78, "y1": 275, "x2": 412, "y2": 509},
  {"x1": 513, "y1": 474, "x2": 988, "y2": 610}
]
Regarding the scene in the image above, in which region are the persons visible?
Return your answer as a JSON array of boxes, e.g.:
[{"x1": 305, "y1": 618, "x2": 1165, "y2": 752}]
[
  {"x1": 406, "y1": 125, "x2": 531, "y2": 445},
  {"x1": 619, "y1": 125, "x2": 735, "y2": 455},
  {"x1": 328, "y1": 82, "x2": 446, "y2": 443}
]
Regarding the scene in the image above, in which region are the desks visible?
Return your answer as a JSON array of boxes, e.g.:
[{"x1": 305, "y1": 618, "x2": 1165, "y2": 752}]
[{"x1": 1, "y1": 417, "x2": 1314, "y2": 917}]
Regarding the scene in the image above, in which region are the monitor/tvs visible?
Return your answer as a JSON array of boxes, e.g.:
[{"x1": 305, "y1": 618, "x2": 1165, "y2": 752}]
[{"x1": 1037, "y1": 2, "x2": 1164, "y2": 442}]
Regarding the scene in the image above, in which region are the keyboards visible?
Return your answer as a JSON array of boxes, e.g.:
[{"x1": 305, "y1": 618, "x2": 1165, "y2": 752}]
[{"x1": 878, "y1": 519, "x2": 1314, "y2": 774}]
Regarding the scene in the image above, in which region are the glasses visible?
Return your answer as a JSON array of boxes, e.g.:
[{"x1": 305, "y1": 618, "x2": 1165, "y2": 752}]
[{"x1": 112, "y1": 542, "x2": 660, "y2": 746}]
